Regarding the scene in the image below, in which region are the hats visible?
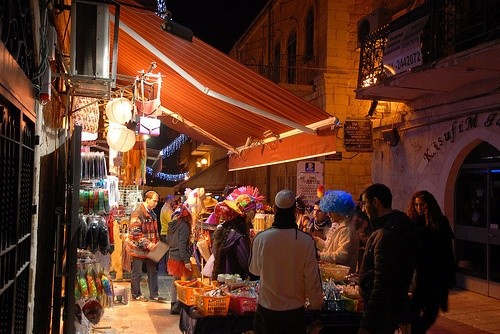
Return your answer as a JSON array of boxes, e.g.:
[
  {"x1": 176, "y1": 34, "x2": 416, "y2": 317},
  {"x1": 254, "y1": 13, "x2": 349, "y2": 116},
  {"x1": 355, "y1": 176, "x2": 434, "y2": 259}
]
[
  {"x1": 274, "y1": 189, "x2": 295, "y2": 209},
  {"x1": 319, "y1": 191, "x2": 355, "y2": 213},
  {"x1": 235, "y1": 193, "x2": 257, "y2": 213},
  {"x1": 170, "y1": 200, "x2": 194, "y2": 222}
]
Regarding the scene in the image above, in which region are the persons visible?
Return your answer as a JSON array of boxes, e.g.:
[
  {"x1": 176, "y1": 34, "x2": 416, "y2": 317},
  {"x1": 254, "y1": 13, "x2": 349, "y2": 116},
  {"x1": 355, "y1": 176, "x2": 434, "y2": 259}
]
[
  {"x1": 407, "y1": 190, "x2": 470, "y2": 334},
  {"x1": 127, "y1": 190, "x2": 168, "y2": 302},
  {"x1": 358, "y1": 183, "x2": 405, "y2": 334},
  {"x1": 167, "y1": 206, "x2": 194, "y2": 313},
  {"x1": 152, "y1": 190, "x2": 369, "y2": 279},
  {"x1": 251, "y1": 189, "x2": 324, "y2": 334}
]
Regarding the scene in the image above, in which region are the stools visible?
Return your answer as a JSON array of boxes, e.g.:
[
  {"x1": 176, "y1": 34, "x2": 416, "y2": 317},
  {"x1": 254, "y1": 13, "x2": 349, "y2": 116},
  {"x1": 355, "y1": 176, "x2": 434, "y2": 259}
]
[{"x1": 113, "y1": 286, "x2": 128, "y2": 304}]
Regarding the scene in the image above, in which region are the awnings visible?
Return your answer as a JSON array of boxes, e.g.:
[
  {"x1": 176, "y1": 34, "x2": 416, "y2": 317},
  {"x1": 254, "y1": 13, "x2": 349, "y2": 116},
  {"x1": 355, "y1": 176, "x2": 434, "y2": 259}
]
[{"x1": 66, "y1": 0, "x2": 337, "y2": 171}]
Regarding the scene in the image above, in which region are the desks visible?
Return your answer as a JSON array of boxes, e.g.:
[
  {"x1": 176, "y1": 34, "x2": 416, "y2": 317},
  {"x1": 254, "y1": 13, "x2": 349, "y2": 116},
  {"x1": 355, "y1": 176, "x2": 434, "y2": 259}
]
[{"x1": 179, "y1": 309, "x2": 364, "y2": 334}]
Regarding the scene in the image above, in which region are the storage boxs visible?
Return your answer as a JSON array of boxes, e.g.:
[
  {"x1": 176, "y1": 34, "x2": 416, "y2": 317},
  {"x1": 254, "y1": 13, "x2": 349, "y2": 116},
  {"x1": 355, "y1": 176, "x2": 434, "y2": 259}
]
[
  {"x1": 316, "y1": 262, "x2": 350, "y2": 282},
  {"x1": 193, "y1": 286, "x2": 230, "y2": 315},
  {"x1": 225, "y1": 290, "x2": 259, "y2": 312},
  {"x1": 174, "y1": 278, "x2": 212, "y2": 306}
]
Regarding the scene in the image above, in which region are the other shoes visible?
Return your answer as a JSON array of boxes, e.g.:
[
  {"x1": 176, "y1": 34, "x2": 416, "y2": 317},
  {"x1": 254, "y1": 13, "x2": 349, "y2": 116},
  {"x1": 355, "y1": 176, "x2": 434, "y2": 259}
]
[
  {"x1": 168, "y1": 300, "x2": 184, "y2": 315},
  {"x1": 133, "y1": 296, "x2": 149, "y2": 302},
  {"x1": 150, "y1": 296, "x2": 167, "y2": 303}
]
